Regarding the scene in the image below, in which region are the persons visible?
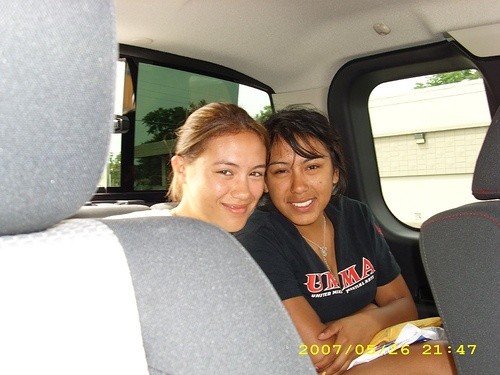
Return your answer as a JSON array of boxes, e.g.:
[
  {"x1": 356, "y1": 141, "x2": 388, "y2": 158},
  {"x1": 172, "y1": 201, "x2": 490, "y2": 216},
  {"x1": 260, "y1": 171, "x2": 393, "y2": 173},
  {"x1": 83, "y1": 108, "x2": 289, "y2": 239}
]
[
  {"x1": 229, "y1": 102, "x2": 458, "y2": 375},
  {"x1": 104, "y1": 101, "x2": 269, "y2": 232}
]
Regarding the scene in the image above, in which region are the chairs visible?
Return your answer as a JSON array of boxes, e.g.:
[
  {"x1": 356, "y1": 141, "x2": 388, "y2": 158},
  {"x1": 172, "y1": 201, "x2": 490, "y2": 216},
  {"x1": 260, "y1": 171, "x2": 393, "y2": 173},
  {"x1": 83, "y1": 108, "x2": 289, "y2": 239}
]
[
  {"x1": 0, "y1": 1, "x2": 318, "y2": 375},
  {"x1": 419, "y1": 104, "x2": 500, "y2": 375}
]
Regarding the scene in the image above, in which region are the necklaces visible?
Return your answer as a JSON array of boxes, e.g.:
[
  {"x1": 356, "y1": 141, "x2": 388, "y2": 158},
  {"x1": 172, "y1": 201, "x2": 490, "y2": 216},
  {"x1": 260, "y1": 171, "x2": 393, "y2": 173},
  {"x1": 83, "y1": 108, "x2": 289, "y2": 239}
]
[{"x1": 302, "y1": 212, "x2": 333, "y2": 274}]
